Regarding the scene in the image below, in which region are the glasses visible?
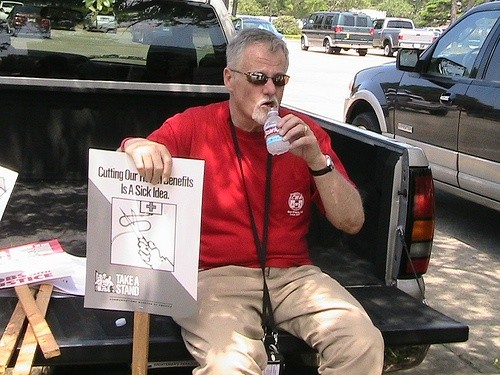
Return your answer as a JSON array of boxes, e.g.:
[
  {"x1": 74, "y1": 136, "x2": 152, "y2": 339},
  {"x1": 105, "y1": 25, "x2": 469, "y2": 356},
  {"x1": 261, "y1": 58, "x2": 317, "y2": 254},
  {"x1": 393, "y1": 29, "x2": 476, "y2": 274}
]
[{"x1": 229, "y1": 67, "x2": 290, "y2": 86}]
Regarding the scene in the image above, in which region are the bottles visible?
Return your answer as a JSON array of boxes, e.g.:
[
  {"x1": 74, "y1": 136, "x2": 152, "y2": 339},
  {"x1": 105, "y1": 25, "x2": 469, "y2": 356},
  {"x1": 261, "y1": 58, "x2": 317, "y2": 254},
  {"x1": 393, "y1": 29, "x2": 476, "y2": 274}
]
[{"x1": 264, "y1": 107, "x2": 290, "y2": 155}]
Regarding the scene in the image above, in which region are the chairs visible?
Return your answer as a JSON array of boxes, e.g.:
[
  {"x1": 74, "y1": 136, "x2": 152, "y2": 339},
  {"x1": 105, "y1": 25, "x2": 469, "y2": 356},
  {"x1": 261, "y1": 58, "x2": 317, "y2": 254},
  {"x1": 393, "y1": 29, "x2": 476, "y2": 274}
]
[{"x1": 146, "y1": 36, "x2": 198, "y2": 83}]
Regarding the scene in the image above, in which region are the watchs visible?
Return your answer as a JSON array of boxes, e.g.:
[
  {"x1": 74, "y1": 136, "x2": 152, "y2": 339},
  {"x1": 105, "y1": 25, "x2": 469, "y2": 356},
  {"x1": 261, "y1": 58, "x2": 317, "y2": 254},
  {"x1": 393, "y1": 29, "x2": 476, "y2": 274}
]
[{"x1": 308, "y1": 154, "x2": 335, "y2": 177}]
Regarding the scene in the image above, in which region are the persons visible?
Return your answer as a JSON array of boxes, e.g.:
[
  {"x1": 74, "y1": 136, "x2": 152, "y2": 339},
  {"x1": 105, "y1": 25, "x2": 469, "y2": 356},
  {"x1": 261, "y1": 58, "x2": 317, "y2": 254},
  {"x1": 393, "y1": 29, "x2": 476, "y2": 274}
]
[{"x1": 114, "y1": 25, "x2": 386, "y2": 375}]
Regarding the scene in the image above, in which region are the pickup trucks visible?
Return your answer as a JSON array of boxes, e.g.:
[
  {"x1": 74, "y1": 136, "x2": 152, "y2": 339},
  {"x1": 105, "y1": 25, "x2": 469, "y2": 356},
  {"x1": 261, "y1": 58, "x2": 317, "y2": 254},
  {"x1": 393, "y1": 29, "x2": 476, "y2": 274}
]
[
  {"x1": 371, "y1": 17, "x2": 441, "y2": 58},
  {"x1": 0, "y1": 0, "x2": 470, "y2": 375}
]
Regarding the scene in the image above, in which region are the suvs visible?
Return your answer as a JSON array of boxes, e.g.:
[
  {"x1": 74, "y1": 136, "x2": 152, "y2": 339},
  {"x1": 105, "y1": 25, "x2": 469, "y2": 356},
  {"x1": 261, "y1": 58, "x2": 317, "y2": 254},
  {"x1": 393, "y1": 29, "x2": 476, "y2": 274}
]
[
  {"x1": 300, "y1": 10, "x2": 376, "y2": 56},
  {"x1": 343, "y1": 0, "x2": 500, "y2": 215}
]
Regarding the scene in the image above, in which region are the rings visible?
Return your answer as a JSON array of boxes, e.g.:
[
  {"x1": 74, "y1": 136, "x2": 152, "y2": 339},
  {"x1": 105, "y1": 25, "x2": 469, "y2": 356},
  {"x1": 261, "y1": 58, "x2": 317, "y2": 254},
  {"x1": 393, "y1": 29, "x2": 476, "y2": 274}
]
[{"x1": 304, "y1": 125, "x2": 308, "y2": 132}]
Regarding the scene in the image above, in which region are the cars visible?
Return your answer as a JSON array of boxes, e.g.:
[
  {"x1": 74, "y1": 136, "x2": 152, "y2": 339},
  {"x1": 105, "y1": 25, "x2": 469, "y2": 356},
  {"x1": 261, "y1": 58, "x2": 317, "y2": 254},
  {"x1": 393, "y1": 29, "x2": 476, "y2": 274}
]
[
  {"x1": 83, "y1": 14, "x2": 118, "y2": 33},
  {"x1": 232, "y1": 16, "x2": 284, "y2": 41},
  {"x1": 5, "y1": 4, "x2": 53, "y2": 39},
  {"x1": 0, "y1": 0, "x2": 27, "y2": 29},
  {"x1": 129, "y1": 19, "x2": 193, "y2": 45}
]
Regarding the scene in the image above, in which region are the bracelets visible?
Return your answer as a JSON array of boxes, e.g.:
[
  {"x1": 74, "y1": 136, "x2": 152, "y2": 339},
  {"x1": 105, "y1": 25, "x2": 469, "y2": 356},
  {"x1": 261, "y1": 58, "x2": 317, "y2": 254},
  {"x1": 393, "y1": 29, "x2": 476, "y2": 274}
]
[{"x1": 120, "y1": 137, "x2": 131, "y2": 152}]
